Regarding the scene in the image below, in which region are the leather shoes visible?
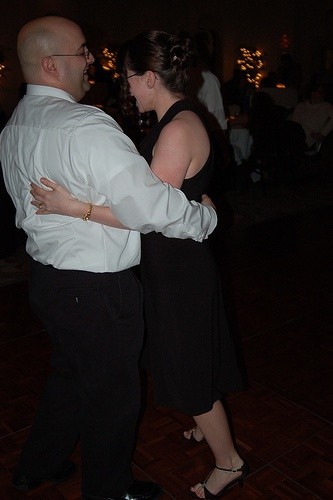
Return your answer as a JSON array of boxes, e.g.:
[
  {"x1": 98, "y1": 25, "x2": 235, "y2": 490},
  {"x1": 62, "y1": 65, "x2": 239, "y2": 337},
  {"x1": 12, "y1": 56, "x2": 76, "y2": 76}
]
[
  {"x1": 82, "y1": 480, "x2": 163, "y2": 500},
  {"x1": 12, "y1": 460, "x2": 76, "y2": 490}
]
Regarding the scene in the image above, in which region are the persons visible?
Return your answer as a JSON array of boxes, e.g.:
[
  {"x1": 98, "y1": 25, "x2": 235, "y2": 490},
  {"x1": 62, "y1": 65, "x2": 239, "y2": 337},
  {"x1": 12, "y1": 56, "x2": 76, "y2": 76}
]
[
  {"x1": 191, "y1": 29, "x2": 230, "y2": 132},
  {"x1": 31, "y1": 33, "x2": 251, "y2": 500},
  {"x1": 0, "y1": 16, "x2": 218, "y2": 500},
  {"x1": 291, "y1": 88, "x2": 333, "y2": 155}
]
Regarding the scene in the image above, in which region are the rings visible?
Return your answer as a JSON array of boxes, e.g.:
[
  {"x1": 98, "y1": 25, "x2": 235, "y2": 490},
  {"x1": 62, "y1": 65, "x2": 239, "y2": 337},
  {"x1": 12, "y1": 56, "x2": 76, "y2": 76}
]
[{"x1": 37, "y1": 202, "x2": 42, "y2": 208}]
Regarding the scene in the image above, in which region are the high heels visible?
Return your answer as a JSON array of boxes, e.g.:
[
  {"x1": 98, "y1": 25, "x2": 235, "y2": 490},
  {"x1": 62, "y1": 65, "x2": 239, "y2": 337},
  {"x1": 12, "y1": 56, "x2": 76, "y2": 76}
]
[
  {"x1": 183, "y1": 429, "x2": 208, "y2": 447},
  {"x1": 188, "y1": 462, "x2": 250, "y2": 500}
]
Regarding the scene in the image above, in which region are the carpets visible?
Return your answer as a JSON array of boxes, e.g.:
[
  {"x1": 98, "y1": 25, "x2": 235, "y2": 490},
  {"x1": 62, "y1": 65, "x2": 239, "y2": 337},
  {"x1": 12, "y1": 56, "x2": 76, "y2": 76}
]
[{"x1": 0, "y1": 164, "x2": 333, "y2": 344}]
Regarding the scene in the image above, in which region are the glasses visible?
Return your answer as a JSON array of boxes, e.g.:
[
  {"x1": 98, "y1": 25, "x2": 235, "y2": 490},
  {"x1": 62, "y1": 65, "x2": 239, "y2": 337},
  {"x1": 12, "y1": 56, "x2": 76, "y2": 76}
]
[
  {"x1": 48, "y1": 51, "x2": 90, "y2": 60},
  {"x1": 120, "y1": 71, "x2": 158, "y2": 84}
]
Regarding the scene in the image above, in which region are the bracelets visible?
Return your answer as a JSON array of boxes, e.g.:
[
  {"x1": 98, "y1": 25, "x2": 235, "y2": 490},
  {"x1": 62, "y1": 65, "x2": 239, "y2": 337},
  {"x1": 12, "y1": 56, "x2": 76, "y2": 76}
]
[{"x1": 82, "y1": 203, "x2": 93, "y2": 221}]
